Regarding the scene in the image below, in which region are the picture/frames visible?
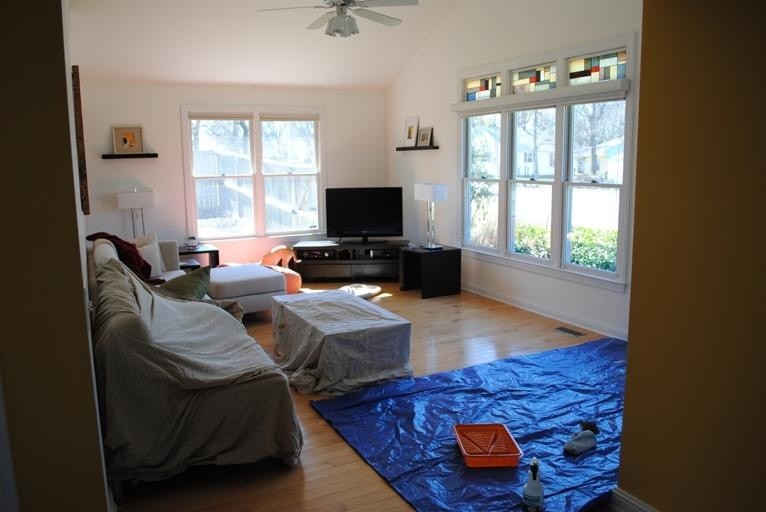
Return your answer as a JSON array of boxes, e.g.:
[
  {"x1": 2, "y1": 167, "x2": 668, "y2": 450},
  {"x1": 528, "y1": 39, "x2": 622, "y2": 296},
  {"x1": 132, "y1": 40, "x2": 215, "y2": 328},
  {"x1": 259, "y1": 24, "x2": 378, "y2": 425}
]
[
  {"x1": 401, "y1": 117, "x2": 418, "y2": 147},
  {"x1": 417, "y1": 127, "x2": 433, "y2": 146},
  {"x1": 112, "y1": 123, "x2": 145, "y2": 153}
]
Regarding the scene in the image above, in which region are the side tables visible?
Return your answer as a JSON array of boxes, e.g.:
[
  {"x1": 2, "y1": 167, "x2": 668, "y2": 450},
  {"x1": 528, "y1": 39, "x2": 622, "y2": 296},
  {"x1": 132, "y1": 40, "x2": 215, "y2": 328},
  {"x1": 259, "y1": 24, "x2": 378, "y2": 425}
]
[{"x1": 179, "y1": 243, "x2": 219, "y2": 268}]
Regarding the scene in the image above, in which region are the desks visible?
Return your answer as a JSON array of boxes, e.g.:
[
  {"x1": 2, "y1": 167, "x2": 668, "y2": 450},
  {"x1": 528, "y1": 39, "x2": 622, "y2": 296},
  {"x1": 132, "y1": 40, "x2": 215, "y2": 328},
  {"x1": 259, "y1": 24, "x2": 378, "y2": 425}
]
[
  {"x1": 292, "y1": 240, "x2": 410, "y2": 282},
  {"x1": 399, "y1": 244, "x2": 461, "y2": 299}
]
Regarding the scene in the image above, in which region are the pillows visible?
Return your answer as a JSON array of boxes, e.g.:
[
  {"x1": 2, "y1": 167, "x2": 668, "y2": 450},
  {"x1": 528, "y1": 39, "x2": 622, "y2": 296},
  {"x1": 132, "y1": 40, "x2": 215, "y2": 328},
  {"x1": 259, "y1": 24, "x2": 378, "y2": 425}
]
[
  {"x1": 137, "y1": 241, "x2": 160, "y2": 277},
  {"x1": 159, "y1": 266, "x2": 210, "y2": 301},
  {"x1": 129, "y1": 233, "x2": 166, "y2": 272}
]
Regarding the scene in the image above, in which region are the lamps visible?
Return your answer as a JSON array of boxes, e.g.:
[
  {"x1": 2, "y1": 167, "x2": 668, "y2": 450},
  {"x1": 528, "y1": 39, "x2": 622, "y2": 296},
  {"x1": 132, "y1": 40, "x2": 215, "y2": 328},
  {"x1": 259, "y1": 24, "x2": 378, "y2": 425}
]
[
  {"x1": 414, "y1": 182, "x2": 447, "y2": 249},
  {"x1": 324, "y1": 6, "x2": 360, "y2": 37},
  {"x1": 116, "y1": 191, "x2": 153, "y2": 239}
]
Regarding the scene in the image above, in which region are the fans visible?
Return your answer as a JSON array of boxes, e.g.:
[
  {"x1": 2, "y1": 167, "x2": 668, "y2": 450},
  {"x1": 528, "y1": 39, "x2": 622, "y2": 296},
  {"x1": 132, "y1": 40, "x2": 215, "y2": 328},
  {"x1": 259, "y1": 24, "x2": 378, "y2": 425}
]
[{"x1": 256, "y1": 0, "x2": 421, "y2": 31}]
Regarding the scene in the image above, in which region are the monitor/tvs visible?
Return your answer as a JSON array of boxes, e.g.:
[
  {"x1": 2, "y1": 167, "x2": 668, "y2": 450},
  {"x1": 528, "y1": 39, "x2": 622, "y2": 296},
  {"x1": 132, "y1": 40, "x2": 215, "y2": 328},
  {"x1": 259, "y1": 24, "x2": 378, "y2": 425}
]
[{"x1": 325, "y1": 187, "x2": 403, "y2": 245}]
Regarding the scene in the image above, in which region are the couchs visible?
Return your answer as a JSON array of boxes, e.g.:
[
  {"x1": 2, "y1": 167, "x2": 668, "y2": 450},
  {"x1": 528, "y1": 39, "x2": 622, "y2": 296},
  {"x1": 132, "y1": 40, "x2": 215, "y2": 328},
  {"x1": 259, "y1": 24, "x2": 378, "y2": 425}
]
[{"x1": 93, "y1": 232, "x2": 303, "y2": 482}]
